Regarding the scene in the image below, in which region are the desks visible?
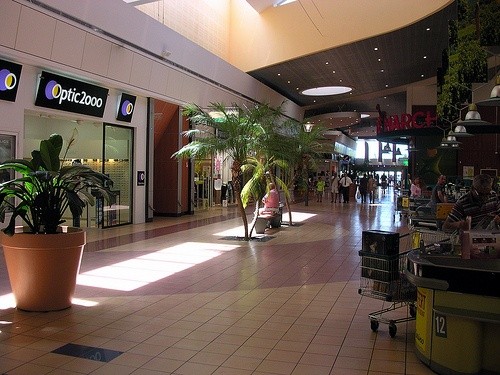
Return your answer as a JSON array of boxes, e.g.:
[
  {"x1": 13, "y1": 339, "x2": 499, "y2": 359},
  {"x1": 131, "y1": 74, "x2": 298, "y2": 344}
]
[{"x1": 86, "y1": 190, "x2": 121, "y2": 228}]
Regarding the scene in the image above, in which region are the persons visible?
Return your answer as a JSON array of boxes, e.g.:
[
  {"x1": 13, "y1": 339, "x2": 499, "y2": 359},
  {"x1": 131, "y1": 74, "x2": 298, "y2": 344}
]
[
  {"x1": 442, "y1": 174, "x2": 500, "y2": 243},
  {"x1": 254, "y1": 183, "x2": 279, "y2": 231},
  {"x1": 431, "y1": 174, "x2": 449, "y2": 230},
  {"x1": 316, "y1": 177, "x2": 324, "y2": 202},
  {"x1": 359, "y1": 173, "x2": 393, "y2": 204},
  {"x1": 394, "y1": 173, "x2": 425, "y2": 201},
  {"x1": 331, "y1": 172, "x2": 353, "y2": 203}
]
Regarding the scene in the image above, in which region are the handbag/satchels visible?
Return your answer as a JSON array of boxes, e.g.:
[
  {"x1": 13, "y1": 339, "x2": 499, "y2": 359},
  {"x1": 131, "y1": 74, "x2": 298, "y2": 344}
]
[{"x1": 357, "y1": 190, "x2": 361, "y2": 199}]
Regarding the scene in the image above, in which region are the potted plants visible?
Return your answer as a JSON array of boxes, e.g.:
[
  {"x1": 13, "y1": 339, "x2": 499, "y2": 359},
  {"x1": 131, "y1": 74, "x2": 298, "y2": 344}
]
[{"x1": 0, "y1": 127, "x2": 114, "y2": 310}]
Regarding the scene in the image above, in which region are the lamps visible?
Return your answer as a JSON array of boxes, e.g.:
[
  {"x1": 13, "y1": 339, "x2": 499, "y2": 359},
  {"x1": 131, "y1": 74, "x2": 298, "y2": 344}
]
[
  {"x1": 448, "y1": 143, "x2": 463, "y2": 149},
  {"x1": 435, "y1": 130, "x2": 451, "y2": 148},
  {"x1": 454, "y1": 91, "x2": 492, "y2": 126},
  {"x1": 446, "y1": 110, "x2": 475, "y2": 137},
  {"x1": 440, "y1": 123, "x2": 461, "y2": 144},
  {"x1": 477, "y1": 74, "x2": 500, "y2": 107}
]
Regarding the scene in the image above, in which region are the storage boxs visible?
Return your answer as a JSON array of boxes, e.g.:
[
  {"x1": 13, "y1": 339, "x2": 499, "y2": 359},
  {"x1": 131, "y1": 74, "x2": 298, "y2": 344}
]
[
  {"x1": 461, "y1": 216, "x2": 500, "y2": 260},
  {"x1": 436, "y1": 203, "x2": 454, "y2": 220},
  {"x1": 360, "y1": 229, "x2": 400, "y2": 282}
]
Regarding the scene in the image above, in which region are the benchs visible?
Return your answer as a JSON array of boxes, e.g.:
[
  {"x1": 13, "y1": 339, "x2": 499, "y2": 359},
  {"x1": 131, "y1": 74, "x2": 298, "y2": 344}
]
[{"x1": 252, "y1": 214, "x2": 281, "y2": 234}]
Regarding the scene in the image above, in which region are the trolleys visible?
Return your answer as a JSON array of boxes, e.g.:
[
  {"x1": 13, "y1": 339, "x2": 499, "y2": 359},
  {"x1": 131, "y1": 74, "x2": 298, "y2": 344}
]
[{"x1": 357, "y1": 227, "x2": 461, "y2": 337}]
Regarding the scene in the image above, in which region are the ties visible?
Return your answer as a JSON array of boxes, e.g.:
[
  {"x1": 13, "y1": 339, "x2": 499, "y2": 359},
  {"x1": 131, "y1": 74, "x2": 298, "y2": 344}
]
[{"x1": 345, "y1": 177, "x2": 346, "y2": 185}]
